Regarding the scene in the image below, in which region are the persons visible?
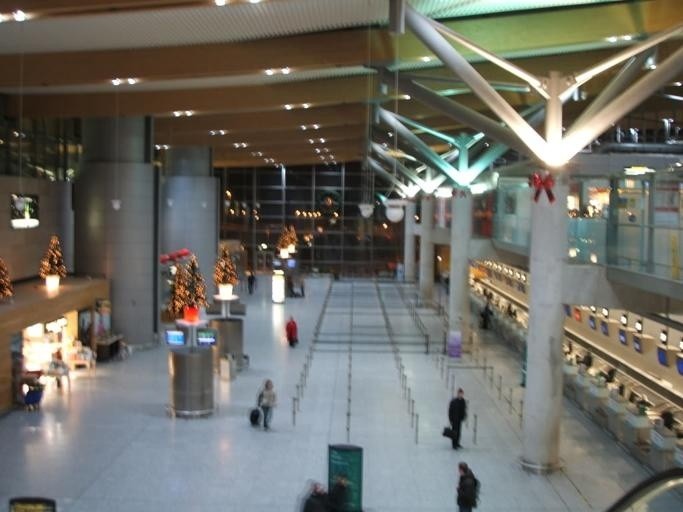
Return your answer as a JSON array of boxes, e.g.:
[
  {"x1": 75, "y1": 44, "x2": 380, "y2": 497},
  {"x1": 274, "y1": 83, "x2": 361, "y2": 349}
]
[
  {"x1": 456, "y1": 462, "x2": 476, "y2": 512},
  {"x1": 286, "y1": 317, "x2": 297, "y2": 346},
  {"x1": 258, "y1": 379, "x2": 276, "y2": 430},
  {"x1": 449, "y1": 388, "x2": 466, "y2": 450},
  {"x1": 248, "y1": 272, "x2": 255, "y2": 295},
  {"x1": 329, "y1": 474, "x2": 353, "y2": 512},
  {"x1": 303, "y1": 482, "x2": 330, "y2": 512}
]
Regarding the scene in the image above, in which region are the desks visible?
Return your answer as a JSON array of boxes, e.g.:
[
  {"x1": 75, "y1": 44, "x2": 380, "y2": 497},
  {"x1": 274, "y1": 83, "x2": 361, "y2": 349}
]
[
  {"x1": 24, "y1": 363, "x2": 70, "y2": 396},
  {"x1": 66, "y1": 352, "x2": 98, "y2": 379}
]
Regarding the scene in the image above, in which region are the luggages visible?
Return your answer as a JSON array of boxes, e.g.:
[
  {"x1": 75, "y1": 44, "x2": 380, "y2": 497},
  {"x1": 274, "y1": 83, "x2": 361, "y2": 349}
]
[{"x1": 250, "y1": 409, "x2": 259, "y2": 426}]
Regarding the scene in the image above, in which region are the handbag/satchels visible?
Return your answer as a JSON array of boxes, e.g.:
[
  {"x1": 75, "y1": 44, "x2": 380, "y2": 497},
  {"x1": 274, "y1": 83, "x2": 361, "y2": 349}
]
[{"x1": 443, "y1": 428, "x2": 457, "y2": 440}]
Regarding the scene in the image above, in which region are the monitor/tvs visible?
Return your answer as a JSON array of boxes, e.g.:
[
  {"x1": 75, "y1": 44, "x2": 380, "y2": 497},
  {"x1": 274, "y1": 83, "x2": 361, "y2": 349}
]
[
  {"x1": 230, "y1": 304, "x2": 246, "y2": 315},
  {"x1": 206, "y1": 303, "x2": 221, "y2": 315},
  {"x1": 196, "y1": 328, "x2": 217, "y2": 345},
  {"x1": 165, "y1": 329, "x2": 186, "y2": 346}
]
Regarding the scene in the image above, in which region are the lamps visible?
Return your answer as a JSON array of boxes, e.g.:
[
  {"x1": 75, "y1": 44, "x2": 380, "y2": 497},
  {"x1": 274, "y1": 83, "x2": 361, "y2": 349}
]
[
  {"x1": 380, "y1": 195, "x2": 410, "y2": 223},
  {"x1": 358, "y1": 202, "x2": 375, "y2": 219}
]
